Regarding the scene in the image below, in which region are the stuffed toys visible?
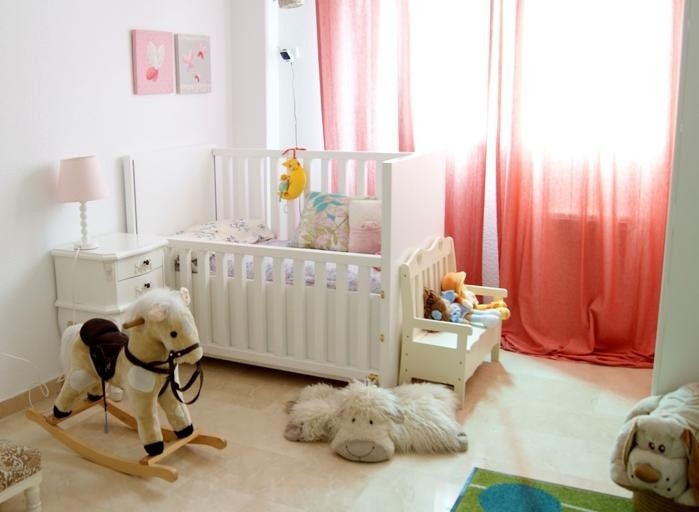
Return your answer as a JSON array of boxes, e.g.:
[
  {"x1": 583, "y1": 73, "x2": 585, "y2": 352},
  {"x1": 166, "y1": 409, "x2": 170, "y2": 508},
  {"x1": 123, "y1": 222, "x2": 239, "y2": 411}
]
[
  {"x1": 610, "y1": 383, "x2": 699, "y2": 507},
  {"x1": 276, "y1": 147, "x2": 306, "y2": 199},
  {"x1": 441, "y1": 271, "x2": 510, "y2": 320},
  {"x1": 283, "y1": 376, "x2": 468, "y2": 462},
  {"x1": 442, "y1": 290, "x2": 501, "y2": 327},
  {"x1": 424, "y1": 288, "x2": 450, "y2": 320}
]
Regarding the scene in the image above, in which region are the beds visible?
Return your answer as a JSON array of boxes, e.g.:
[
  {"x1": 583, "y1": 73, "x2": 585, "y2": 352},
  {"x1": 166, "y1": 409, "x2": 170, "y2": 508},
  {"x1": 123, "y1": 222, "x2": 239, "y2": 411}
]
[{"x1": 124, "y1": 144, "x2": 443, "y2": 391}]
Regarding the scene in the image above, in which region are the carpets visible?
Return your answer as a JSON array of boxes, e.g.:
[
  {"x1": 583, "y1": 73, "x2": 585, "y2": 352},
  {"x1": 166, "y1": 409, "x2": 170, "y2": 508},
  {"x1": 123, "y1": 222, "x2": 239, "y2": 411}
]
[{"x1": 451, "y1": 468, "x2": 634, "y2": 511}]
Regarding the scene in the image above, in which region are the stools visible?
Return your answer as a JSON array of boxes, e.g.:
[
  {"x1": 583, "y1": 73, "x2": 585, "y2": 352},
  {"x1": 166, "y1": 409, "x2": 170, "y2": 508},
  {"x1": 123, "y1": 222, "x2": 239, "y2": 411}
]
[{"x1": 0, "y1": 440, "x2": 42, "y2": 511}]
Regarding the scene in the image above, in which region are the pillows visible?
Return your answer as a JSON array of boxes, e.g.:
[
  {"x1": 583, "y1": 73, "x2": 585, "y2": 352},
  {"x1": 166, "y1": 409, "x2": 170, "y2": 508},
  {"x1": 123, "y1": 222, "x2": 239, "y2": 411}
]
[{"x1": 171, "y1": 217, "x2": 276, "y2": 274}]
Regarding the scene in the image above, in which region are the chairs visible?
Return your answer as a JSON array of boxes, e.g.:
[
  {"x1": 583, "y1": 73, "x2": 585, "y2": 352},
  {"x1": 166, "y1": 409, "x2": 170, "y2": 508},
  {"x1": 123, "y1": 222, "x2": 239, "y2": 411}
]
[{"x1": 399, "y1": 234, "x2": 507, "y2": 411}]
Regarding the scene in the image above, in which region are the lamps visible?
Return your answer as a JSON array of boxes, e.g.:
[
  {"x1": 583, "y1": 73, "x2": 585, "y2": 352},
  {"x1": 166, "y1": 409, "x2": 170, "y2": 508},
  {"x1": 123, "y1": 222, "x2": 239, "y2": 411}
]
[{"x1": 55, "y1": 156, "x2": 108, "y2": 251}]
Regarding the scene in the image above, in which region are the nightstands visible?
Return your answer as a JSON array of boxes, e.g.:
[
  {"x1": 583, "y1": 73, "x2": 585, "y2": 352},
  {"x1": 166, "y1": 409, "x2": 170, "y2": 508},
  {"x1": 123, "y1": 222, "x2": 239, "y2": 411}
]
[{"x1": 51, "y1": 233, "x2": 166, "y2": 340}]
